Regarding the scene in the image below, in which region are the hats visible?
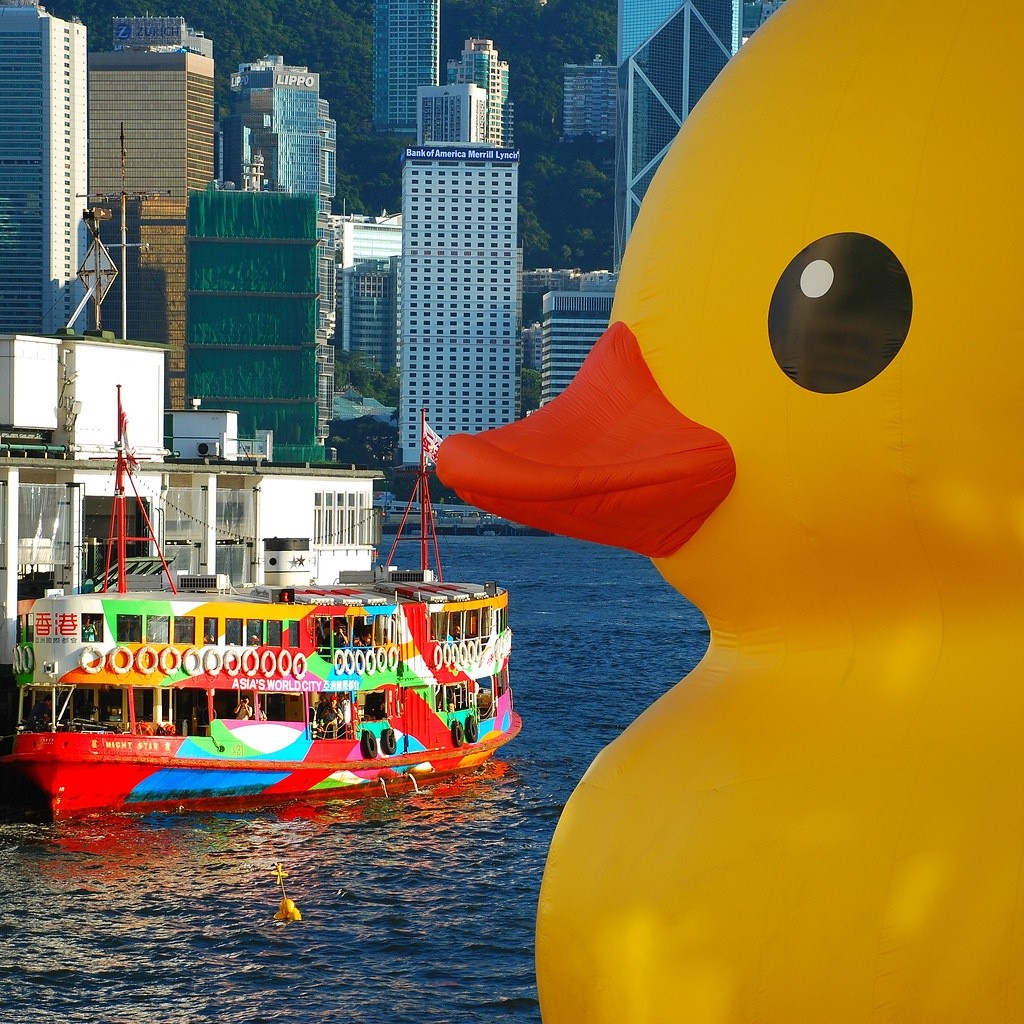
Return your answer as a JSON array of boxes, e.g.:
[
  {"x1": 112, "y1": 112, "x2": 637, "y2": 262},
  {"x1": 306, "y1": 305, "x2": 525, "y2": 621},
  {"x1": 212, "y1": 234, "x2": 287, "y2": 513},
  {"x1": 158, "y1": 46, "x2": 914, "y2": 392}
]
[{"x1": 330, "y1": 695, "x2": 337, "y2": 701}]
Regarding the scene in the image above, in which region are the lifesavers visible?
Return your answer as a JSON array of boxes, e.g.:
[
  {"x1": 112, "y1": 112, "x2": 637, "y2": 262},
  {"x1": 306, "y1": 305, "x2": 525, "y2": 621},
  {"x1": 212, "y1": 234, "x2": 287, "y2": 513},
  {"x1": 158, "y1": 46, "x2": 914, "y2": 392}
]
[
  {"x1": 451, "y1": 721, "x2": 464, "y2": 747},
  {"x1": 361, "y1": 730, "x2": 378, "y2": 759},
  {"x1": 334, "y1": 646, "x2": 399, "y2": 675},
  {"x1": 433, "y1": 637, "x2": 484, "y2": 670},
  {"x1": 494, "y1": 629, "x2": 512, "y2": 660},
  {"x1": 464, "y1": 716, "x2": 479, "y2": 743},
  {"x1": 381, "y1": 728, "x2": 396, "y2": 755},
  {"x1": 77, "y1": 645, "x2": 308, "y2": 680},
  {"x1": 10, "y1": 645, "x2": 34, "y2": 674},
  {"x1": 136, "y1": 722, "x2": 153, "y2": 737},
  {"x1": 156, "y1": 723, "x2": 176, "y2": 736}
]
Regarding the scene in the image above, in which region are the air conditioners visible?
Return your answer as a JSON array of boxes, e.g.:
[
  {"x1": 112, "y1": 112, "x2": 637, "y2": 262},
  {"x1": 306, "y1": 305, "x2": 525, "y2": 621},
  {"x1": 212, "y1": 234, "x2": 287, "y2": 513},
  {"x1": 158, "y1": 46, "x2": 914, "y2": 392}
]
[{"x1": 195, "y1": 442, "x2": 219, "y2": 456}]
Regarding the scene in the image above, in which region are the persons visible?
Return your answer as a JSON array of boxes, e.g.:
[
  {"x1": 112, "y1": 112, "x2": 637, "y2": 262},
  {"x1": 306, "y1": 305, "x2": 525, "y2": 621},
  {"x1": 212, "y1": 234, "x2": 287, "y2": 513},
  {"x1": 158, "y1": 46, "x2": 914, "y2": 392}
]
[
  {"x1": 321, "y1": 626, "x2": 461, "y2": 663},
  {"x1": 30, "y1": 695, "x2": 52, "y2": 722},
  {"x1": 117, "y1": 617, "x2": 136, "y2": 642},
  {"x1": 233, "y1": 695, "x2": 268, "y2": 720},
  {"x1": 201, "y1": 704, "x2": 217, "y2": 725},
  {"x1": 82, "y1": 616, "x2": 98, "y2": 642},
  {"x1": 315, "y1": 691, "x2": 456, "y2": 740},
  {"x1": 180, "y1": 626, "x2": 263, "y2": 646}
]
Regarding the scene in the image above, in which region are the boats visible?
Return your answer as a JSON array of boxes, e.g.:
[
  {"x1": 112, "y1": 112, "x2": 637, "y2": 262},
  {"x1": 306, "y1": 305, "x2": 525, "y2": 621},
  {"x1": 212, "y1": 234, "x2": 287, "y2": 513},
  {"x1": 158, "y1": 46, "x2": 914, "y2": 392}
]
[{"x1": 1, "y1": 384, "x2": 524, "y2": 827}]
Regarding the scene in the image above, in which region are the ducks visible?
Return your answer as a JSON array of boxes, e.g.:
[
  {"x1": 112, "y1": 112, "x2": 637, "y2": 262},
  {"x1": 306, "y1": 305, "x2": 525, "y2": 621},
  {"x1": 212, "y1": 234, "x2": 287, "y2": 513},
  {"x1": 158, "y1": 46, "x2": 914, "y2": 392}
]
[{"x1": 437, "y1": 0, "x2": 1024, "y2": 1024}]
